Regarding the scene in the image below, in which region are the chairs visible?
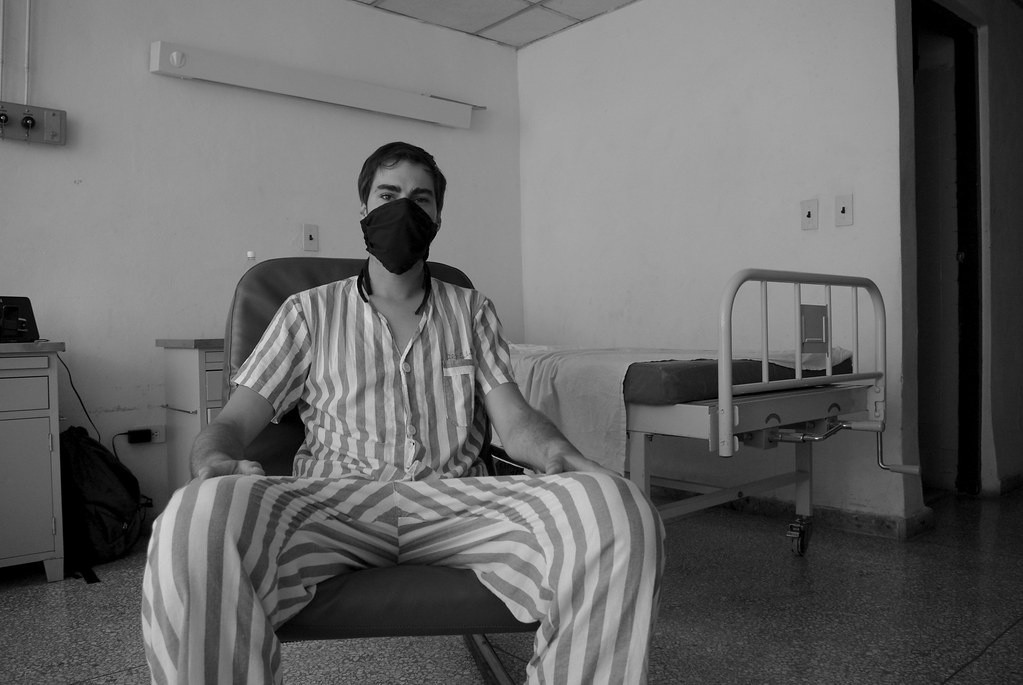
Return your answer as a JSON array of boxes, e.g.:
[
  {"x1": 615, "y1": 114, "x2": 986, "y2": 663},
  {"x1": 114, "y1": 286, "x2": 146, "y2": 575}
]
[{"x1": 221, "y1": 257, "x2": 543, "y2": 685}]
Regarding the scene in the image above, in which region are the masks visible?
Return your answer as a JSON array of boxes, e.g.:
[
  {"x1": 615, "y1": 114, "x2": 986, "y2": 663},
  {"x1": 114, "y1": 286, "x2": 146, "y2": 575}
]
[{"x1": 359, "y1": 198, "x2": 437, "y2": 275}]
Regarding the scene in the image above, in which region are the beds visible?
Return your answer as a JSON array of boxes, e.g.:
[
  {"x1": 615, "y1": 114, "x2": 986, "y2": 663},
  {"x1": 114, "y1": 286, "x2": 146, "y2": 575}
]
[{"x1": 488, "y1": 267, "x2": 923, "y2": 559}]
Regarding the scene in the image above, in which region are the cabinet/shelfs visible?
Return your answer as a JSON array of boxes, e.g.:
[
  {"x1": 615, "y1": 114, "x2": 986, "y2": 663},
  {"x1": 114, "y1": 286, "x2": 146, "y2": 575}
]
[
  {"x1": 156, "y1": 337, "x2": 227, "y2": 497},
  {"x1": 0, "y1": 341, "x2": 66, "y2": 582}
]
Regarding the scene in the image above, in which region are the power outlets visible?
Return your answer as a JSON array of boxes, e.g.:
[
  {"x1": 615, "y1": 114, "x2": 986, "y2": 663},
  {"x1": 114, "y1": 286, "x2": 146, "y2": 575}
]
[{"x1": 151, "y1": 426, "x2": 167, "y2": 443}]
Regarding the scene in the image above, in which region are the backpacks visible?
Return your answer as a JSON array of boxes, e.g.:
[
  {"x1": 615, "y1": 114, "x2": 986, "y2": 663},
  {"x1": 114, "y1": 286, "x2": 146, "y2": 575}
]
[{"x1": 60, "y1": 426, "x2": 141, "y2": 585}]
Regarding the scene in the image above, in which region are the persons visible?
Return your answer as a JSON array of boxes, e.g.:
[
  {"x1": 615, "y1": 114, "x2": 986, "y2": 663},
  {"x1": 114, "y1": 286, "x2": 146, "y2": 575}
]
[{"x1": 140, "y1": 141, "x2": 665, "y2": 685}]
